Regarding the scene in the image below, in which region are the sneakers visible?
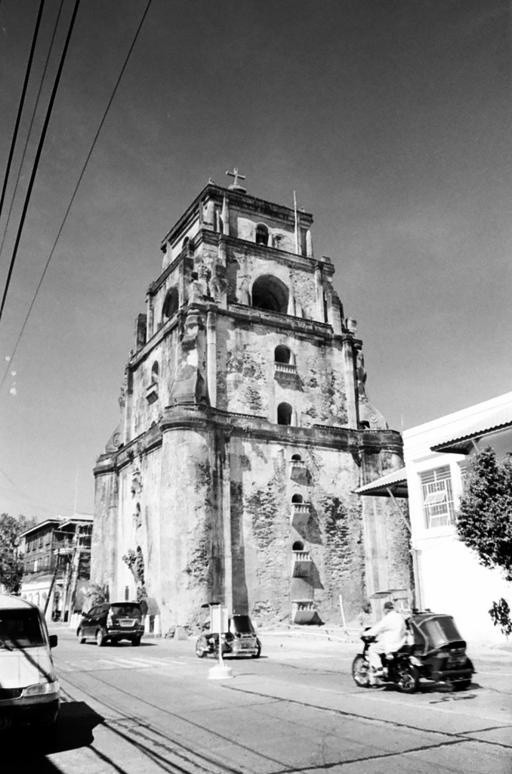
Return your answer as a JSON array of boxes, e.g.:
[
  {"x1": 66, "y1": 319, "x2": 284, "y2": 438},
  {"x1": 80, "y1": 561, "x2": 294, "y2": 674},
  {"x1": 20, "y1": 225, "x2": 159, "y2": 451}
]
[{"x1": 371, "y1": 670, "x2": 384, "y2": 677}]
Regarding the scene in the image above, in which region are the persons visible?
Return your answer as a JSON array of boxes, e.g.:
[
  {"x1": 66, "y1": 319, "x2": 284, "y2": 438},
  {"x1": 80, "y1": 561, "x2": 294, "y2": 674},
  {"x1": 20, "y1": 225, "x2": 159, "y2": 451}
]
[{"x1": 362, "y1": 602, "x2": 410, "y2": 676}]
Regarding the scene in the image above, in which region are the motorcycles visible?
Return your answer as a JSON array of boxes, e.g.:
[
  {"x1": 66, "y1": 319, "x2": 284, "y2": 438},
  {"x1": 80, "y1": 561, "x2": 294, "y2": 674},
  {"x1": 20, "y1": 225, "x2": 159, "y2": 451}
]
[
  {"x1": 195, "y1": 614, "x2": 262, "y2": 657},
  {"x1": 352, "y1": 610, "x2": 479, "y2": 692}
]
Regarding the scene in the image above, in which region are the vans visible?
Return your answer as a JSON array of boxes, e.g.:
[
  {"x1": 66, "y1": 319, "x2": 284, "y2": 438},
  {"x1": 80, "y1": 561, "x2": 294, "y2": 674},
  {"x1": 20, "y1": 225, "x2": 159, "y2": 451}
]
[
  {"x1": 0, "y1": 595, "x2": 61, "y2": 726},
  {"x1": 77, "y1": 602, "x2": 144, "y2": 646}
]
